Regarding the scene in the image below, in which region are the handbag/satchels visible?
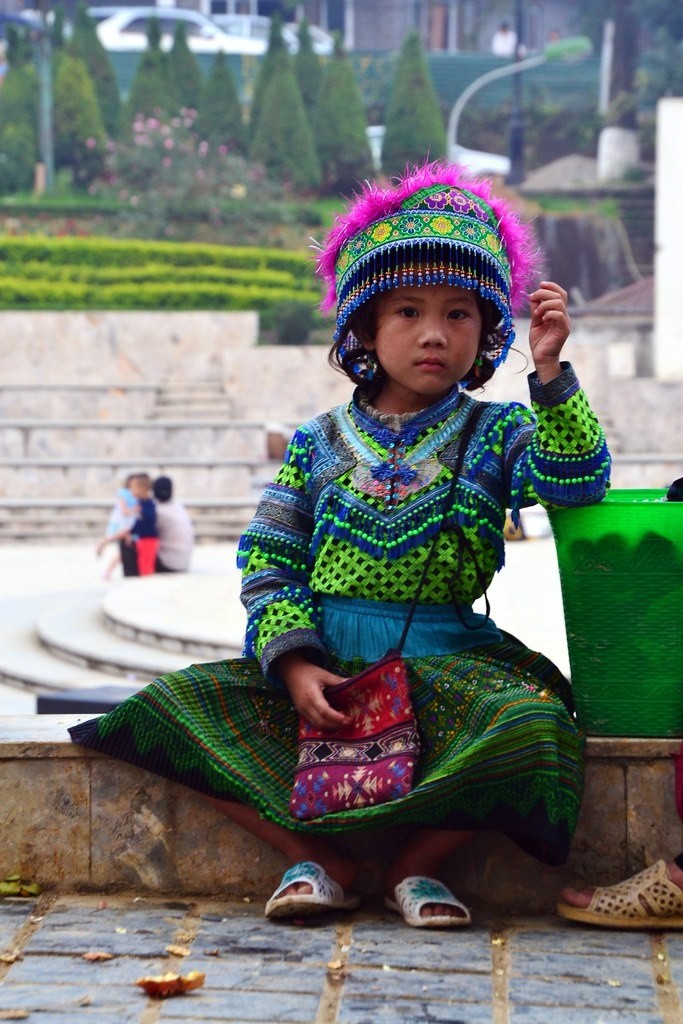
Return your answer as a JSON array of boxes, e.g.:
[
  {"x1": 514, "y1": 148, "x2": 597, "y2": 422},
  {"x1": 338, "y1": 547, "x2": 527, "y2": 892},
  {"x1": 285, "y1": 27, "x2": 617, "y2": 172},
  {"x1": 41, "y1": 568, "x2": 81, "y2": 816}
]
[{"x1": 289, "y1": 648, "x2": 422, "y2": 820}]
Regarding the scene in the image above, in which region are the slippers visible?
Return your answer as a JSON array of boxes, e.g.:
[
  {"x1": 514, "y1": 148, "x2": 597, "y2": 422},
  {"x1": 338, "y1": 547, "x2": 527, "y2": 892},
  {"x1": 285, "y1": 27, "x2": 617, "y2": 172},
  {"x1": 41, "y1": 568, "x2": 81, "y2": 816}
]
[
  {"x1": 385, "y1": 875, "x2": 471, "y2": 927},
  {"x1": 265, "y1": 862, "x2": 359, "y2": 918}
]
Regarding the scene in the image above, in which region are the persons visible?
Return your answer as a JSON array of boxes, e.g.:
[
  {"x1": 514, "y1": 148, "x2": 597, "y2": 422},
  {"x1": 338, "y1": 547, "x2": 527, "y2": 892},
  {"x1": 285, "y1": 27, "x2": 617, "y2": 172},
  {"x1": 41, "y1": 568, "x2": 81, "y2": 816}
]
[
  {"x1": 67, "y1": 159, "x2": 612, "y2": 929},
  {"x1": 556, "y1": 850, "x2": 683, "y2": 927},
  {"x1": 97, "y1": 474, "x2": 194, "y2": 580}
]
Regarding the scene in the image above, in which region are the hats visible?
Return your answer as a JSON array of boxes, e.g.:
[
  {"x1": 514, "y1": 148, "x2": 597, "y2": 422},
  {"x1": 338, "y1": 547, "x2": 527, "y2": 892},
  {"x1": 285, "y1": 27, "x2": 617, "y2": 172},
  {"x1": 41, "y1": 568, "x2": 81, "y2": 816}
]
[{"x1": 305, "y1": 145, "x2": 544, "y2": 390}]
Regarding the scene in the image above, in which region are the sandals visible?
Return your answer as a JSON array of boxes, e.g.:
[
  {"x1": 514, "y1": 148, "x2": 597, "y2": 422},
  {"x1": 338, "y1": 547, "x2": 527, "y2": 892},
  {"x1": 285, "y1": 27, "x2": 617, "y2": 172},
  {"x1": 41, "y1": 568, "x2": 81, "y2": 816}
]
[{"x1": 556, "y1": 859, "x2": 683, "y2": 929}]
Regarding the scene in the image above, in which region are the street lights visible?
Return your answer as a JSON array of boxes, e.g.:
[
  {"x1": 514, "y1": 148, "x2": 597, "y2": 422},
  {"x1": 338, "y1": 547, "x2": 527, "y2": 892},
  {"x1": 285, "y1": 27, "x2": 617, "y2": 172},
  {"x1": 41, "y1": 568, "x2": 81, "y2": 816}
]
[{"x1": 447, "y1": 37, "x2": 595, "y2": 166}]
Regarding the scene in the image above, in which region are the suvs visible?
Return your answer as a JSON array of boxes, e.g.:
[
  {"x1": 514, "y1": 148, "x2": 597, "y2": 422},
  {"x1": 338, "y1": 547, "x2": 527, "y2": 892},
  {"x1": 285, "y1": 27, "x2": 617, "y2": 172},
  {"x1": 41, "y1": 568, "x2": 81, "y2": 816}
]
[{"x1": 66, "y1": 7, "x2": 269, "y2": 56}]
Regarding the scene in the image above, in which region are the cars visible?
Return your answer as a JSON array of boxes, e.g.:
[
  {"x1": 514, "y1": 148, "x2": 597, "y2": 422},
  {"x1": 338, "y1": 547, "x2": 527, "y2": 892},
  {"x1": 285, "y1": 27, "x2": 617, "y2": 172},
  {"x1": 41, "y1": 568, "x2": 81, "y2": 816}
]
[
  {"x1": 211, "y1": 13, "x2": 337, "y2": 54},
  {"x1": 364, "y1": 125, "x2": 512, "y2": 183}
]
